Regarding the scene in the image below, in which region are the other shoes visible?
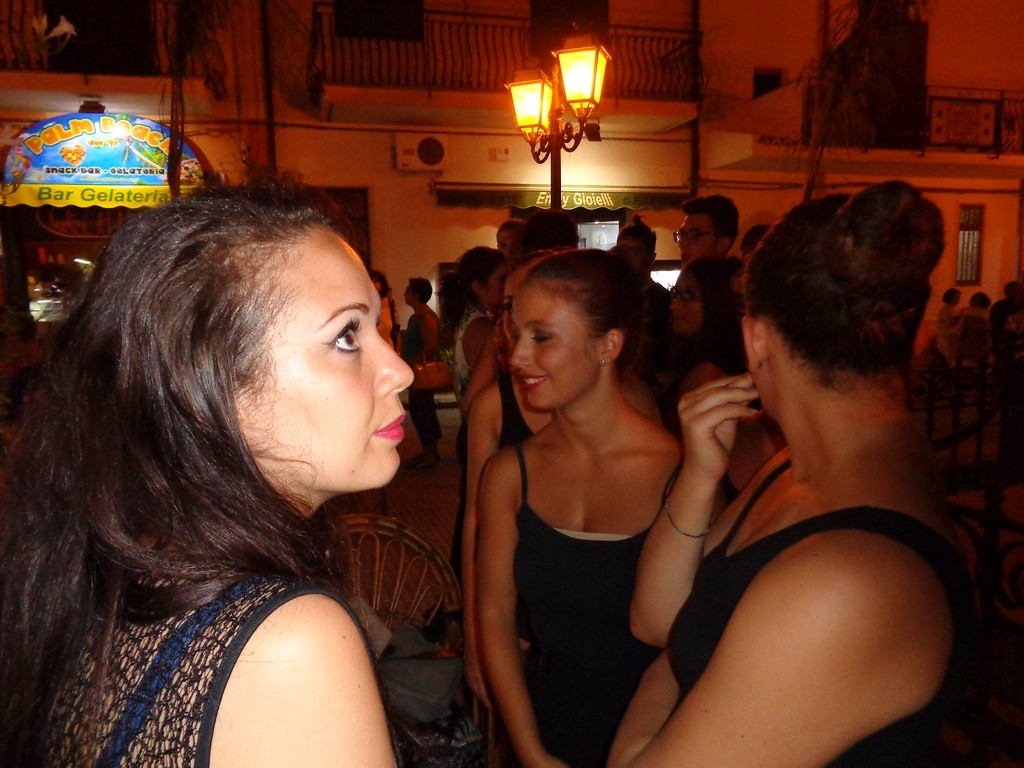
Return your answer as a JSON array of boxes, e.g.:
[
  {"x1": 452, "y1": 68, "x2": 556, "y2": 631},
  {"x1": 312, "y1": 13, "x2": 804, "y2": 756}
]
[{"x1": 406, "y1": 452, "x2": 444, "y2": 467}]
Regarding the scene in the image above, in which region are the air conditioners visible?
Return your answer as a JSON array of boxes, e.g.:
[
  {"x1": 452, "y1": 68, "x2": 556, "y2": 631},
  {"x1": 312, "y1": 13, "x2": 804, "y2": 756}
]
[{"x1": 396, "y1": 132, "x2": 448, "y2": 172}]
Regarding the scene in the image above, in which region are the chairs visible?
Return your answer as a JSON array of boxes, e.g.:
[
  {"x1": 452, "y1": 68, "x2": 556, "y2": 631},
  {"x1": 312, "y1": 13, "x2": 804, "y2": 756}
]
[{"x1": 324, "y1": 514, "x2": 495, "y2": 768}]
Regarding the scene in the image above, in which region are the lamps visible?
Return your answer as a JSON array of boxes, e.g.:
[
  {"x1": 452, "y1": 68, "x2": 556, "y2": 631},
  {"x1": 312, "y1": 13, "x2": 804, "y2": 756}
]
[{"x1": 78, "y1": 94, "x2": 105, "y2": 114}]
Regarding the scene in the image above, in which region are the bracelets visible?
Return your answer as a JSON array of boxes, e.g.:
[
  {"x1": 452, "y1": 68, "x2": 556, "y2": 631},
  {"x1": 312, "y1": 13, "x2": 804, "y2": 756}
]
[{"x1": 665, "y1": 496, "x2": 715, "y2": 537}]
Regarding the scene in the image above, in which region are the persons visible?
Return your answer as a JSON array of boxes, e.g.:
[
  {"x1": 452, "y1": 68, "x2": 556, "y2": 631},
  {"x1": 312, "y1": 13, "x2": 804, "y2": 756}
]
[
  {"x1": 368, "y1": 195, "x2": 773, "y2": 768},
  {"x1": 937, "y1": 281, "x2": 1024, "y2": 423},
  {"x1": 608, "y1": 181, "x2": 971, "y2": 768},
  {"x1": 0, "y1": 169, "x2": 413, "y2": 768},
  {"x1": 44, "y1": 252, "x2": 66, "y2": 282}
]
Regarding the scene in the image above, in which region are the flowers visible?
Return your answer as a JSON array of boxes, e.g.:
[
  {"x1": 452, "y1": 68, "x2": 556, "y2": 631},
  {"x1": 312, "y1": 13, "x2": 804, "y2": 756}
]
[{"x1": 12, "y1": 13, "x2": 78, "y2": 69}]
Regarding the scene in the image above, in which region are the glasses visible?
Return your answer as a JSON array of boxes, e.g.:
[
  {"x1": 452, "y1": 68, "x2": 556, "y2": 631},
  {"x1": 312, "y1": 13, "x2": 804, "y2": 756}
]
[
  {"x1": 670, "y1": 287, "x2": 700, "y2": 302},
  {"x1": 673, "y1": 227, "x2": 718, "y2": 244}
]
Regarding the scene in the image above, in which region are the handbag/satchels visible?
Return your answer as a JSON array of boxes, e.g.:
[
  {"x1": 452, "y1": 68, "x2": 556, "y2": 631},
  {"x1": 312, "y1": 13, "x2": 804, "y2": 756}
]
[{"x1": 412, "y1": 344, "x2": 456, "y2": 393}]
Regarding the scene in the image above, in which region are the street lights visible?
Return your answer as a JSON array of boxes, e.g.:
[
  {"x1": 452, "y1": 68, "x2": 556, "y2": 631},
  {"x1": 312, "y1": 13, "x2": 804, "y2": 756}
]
[{"x1": 503, "y1": 18, "x2": 615, "y2": 210}]
